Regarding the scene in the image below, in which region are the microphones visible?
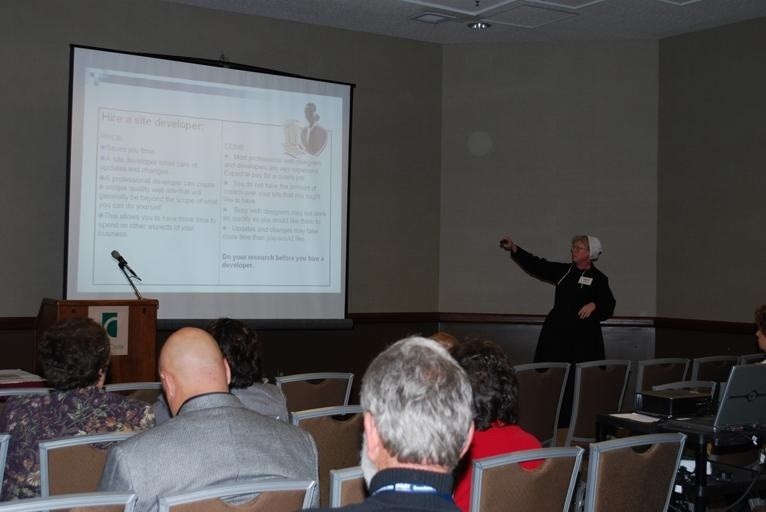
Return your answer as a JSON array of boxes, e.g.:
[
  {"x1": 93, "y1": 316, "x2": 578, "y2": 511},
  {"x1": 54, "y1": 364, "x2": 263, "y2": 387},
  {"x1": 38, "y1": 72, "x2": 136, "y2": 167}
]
[{"x1": 111, "y1": 249, "x2": 141, "y2": 282}]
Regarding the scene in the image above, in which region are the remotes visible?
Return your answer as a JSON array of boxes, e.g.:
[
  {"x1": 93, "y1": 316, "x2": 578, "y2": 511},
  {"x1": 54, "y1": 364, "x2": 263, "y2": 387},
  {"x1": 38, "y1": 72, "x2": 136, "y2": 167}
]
[{"x1": 498, "y1": 238, "x2": 508, "y2": 243}]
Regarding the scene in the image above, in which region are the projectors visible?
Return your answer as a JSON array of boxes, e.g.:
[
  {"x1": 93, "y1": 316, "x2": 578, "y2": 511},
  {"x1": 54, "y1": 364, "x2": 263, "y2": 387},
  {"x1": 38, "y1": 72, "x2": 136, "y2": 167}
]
[{"x1": 633, "y1": 387, "x2": 713, "y2": 419}]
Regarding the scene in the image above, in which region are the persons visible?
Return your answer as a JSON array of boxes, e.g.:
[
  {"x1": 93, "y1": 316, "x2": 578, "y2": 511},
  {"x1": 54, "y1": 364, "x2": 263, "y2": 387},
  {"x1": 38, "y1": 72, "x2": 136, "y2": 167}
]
[
  {"x1": 330, "y1": 334, "x2": 475, "y2": 511},
  {"x1": 427, "y1": 331, "x2": 459, "y2": 349},
  {"x1": 98, "y1": 326, "x2": 320, "y2": 510},
  {"x1": 150, "y1": 315, "x2": 289, "y2": 424},
  {"x1": 446, "y1": 338, "x2": 544, "y2": 511},
  {"x1": 501, "y1": 231, "x2": 619, "y2": 429},
  {"x1": 0, "y1": 316, "x2": 156, "y2": 504},
  {"x1": 752, "y1": 304, "x2": 766, "y2": 366},
  {"x1": 298, "y1": 100, "x2": 328, "y2": 159}
]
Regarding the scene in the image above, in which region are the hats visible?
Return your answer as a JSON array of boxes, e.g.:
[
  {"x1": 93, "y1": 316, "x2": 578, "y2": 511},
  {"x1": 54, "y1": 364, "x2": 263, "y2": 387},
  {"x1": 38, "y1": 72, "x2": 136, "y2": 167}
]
[{"x1": 586, "y1": 234, "x2": 602, "y2": 260}]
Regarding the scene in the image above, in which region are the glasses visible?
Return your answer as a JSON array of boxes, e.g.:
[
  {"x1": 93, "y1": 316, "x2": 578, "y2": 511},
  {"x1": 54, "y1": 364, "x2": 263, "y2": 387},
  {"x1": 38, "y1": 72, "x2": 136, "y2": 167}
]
[{"x1": 571, "y1": 246, "x2": 587, "y2": 253}]
